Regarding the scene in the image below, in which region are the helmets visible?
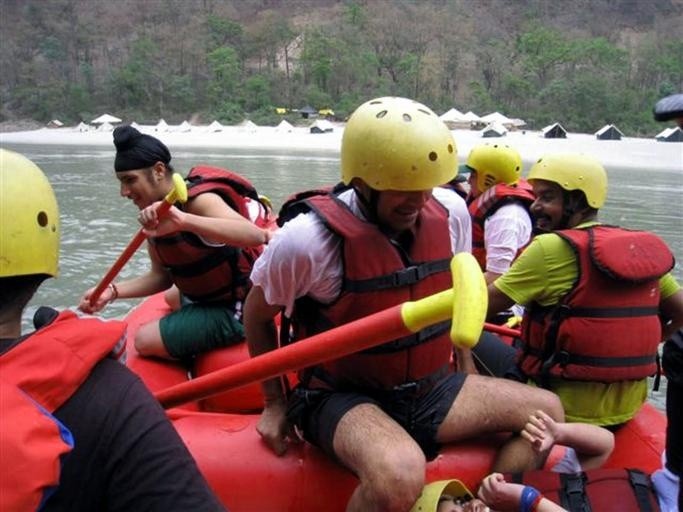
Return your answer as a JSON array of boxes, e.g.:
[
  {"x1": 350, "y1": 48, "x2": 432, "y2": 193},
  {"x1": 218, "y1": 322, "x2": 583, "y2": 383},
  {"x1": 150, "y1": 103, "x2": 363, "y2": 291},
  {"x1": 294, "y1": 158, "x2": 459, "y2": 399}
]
[
  {"x1": 465, "y1": 142, "x2": 522, "y2": 193},
  {"x1": 527, "y1": 153, "x2": 608, "y2": 209},
  {"x1": 408, "y1": 478, "x2": 473, "y2": 512},
  {"x1": 0, "y1": 148, "x2": 62, "y2": 279},
  {"x1": 339, "y1": 96, "x2": 458, "y2": 192}
]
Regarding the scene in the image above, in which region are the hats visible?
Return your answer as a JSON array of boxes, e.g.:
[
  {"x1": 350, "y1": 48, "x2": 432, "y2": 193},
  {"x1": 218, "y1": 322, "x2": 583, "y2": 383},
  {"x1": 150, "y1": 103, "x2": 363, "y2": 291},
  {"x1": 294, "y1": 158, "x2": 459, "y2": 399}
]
[{"x1": 114, "y1": 124, "x2": 172, "y2": 170}]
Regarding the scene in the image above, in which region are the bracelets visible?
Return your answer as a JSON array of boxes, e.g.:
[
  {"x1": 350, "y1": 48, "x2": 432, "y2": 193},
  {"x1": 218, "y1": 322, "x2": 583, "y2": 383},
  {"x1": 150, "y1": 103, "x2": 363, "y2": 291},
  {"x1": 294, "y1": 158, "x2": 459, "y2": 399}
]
[
  {"x1": 108, "y1": 283, "x2": 118, "y2": 301},
  {"x1": 516, "y1": 483, "x2": 544, "y2": 511}
]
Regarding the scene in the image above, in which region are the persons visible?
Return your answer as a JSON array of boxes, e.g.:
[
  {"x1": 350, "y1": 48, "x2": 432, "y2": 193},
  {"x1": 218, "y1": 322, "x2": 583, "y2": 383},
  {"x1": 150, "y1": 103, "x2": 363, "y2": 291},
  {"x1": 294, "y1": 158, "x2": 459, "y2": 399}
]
[
  {"x1": 75, "y1": 124, "x2": 285, "y2": 362},
  {"x1": 396, "y1": 408, "x2": 617, "y2": 511},
  {"x1": 460, "y1": 150, "x2": 682, "y2": 438},
  {"x1": 239, "y1": 94, "x2": 569, "y2": 510},
  {"x1": 459, "y1": 138, "x2": 540, "y2": 290},
  {"x1": 1, "y1": 142, "x2": 231, "y2": 510}
]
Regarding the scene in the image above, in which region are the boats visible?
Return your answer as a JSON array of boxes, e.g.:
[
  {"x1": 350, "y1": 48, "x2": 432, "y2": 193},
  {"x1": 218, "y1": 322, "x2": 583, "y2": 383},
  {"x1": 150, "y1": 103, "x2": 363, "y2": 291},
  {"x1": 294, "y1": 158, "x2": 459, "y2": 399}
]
[{"x1": 118, "y1": 287, "x2": 669, "y2": 512}]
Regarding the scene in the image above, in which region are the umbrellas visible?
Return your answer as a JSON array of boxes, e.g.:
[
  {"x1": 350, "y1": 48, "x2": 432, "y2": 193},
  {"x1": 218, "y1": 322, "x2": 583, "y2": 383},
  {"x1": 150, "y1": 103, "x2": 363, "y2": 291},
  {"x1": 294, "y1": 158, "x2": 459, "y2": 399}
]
[{"x1": 90, "y1": 113, "x2": 123, "y2": 125}]
